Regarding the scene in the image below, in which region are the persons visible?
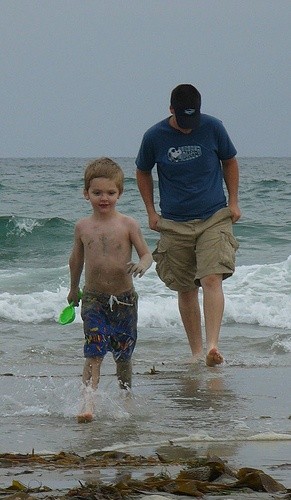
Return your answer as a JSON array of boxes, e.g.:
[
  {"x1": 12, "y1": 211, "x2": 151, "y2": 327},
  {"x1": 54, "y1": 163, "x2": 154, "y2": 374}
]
[
  {"x1": 68, "y1": 156, "x2": 154, "y2": 424},
  {"x1": 134, "y1": 83, "x2": 243, "y2": 368}
]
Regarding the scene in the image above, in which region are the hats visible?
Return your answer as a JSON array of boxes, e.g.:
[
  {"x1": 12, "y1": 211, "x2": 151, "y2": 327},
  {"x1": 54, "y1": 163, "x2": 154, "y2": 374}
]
[{"x1": 171, "y1": 84, "x2": 201, "y2": 128}]
